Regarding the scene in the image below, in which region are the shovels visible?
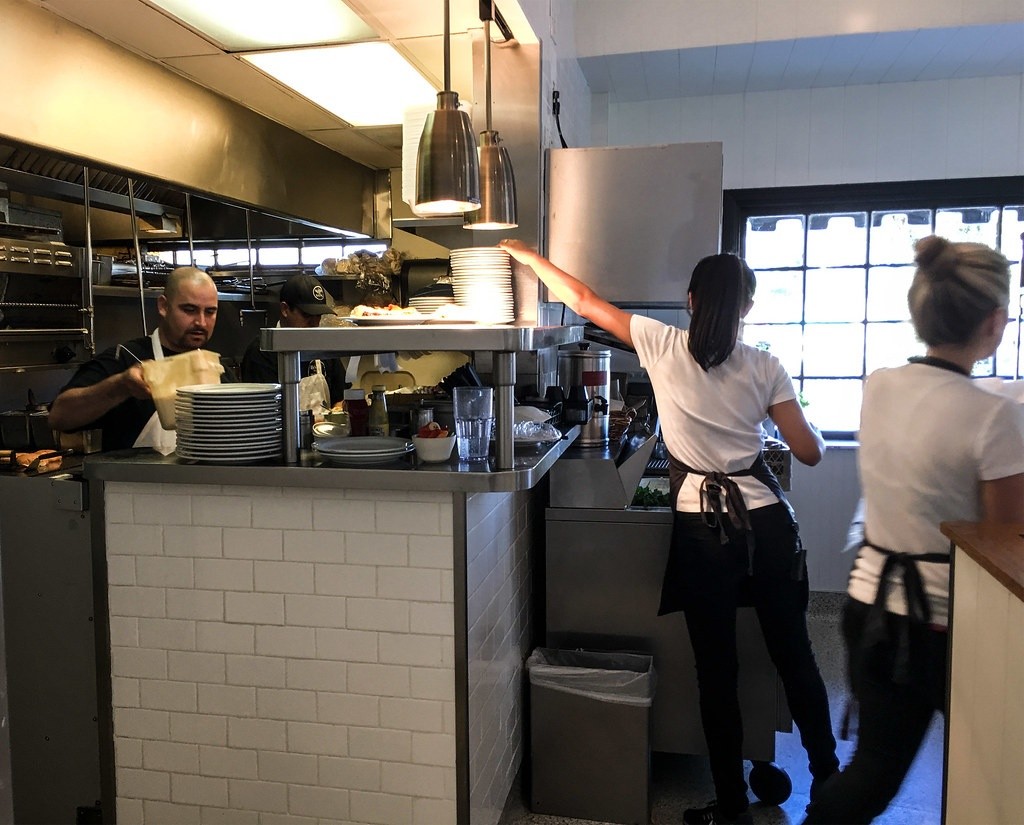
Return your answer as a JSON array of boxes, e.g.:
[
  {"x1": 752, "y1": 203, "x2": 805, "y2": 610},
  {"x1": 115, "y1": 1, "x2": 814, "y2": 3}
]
[{"x1": 27, "y1": 449, "x2": 75, "y2": 472}]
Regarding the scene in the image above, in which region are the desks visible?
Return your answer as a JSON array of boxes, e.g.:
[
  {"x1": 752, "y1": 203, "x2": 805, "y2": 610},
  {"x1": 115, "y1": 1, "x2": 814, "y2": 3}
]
[{"x1": 939, "y1": 522, "x2": 1023, "y2": 825}]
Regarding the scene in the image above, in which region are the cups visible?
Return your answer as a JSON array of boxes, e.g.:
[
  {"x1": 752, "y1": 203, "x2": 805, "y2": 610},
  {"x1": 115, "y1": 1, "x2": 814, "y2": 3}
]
[{"x1": 452, "y1": 387, "x2": 490, "y2": 462}]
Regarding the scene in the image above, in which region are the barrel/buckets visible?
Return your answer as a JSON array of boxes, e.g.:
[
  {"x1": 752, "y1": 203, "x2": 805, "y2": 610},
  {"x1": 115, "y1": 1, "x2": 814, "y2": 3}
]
[{"x1": 556, "y1": 340, "x2": 611, "y2": 450}]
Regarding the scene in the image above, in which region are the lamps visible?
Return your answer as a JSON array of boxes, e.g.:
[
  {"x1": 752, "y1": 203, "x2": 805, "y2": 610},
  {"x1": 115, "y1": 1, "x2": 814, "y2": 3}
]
[
  {"x1": 461, "y1": 20, "x2": 520, "y2": 230},
  {"x1": 414, "y1": 1, "x2": 482, "y2": 218}
]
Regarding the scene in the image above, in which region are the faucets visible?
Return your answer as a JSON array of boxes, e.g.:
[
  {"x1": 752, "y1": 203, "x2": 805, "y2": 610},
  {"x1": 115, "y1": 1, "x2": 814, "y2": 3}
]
[{"x1": 594, "y1": 395, "x2": 611, "y2": 416}]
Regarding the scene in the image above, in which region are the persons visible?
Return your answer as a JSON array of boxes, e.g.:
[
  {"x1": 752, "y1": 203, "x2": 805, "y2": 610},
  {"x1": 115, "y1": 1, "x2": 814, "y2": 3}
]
[
  {"x1": 241, "y1": 274, "x2": 352, "y2": 414},
  {"x1": 801, "y1": 234, "x2": 1024, "y2": 825},
  {"x1": 497, "y1": 237, "x2": 843, "y2": 824},
  {"x1": 46, "y1": 266, "x2": 239, "y2": 455}
]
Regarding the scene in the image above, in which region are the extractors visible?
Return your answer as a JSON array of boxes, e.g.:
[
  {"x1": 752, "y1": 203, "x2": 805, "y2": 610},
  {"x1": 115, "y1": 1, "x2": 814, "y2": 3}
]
[{"x1": 0, "y1": 236, "x2": 89, "y2": 343}]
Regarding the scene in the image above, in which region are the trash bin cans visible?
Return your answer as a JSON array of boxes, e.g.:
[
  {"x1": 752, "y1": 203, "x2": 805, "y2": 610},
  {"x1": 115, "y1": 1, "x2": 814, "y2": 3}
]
[{"x1": 523, "y1": 645, "x2": 658, "y2": 824}]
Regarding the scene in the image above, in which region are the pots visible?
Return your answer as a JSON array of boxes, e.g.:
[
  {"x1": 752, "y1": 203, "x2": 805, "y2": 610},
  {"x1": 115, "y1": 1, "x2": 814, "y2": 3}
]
[{"x1": 3, "y1": 401, "x2": 96, "y2": 456}]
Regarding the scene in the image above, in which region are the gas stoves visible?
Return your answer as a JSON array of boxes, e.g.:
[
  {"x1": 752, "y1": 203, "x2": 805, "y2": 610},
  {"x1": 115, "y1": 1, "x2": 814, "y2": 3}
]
[{"x1": 0, "y1": 436, "x2": 103, "y2": 479}]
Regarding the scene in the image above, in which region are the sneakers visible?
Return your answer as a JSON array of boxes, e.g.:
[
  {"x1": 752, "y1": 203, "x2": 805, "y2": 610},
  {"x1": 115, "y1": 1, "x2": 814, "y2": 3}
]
[{"x1": 682, "y1": 803, "x2": 738, "y2": 825}]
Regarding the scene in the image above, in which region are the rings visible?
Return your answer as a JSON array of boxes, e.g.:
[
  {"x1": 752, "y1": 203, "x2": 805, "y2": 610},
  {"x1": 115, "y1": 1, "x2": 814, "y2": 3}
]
[{"x1": 503, "y1": 239, "x2": 509, "y2": 244}]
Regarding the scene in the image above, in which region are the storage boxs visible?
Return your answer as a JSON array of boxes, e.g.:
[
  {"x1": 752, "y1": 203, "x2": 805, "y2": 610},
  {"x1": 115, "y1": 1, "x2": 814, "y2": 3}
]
[{"x1": 761, "y1": 434, "x2": 792, "y2": 492}]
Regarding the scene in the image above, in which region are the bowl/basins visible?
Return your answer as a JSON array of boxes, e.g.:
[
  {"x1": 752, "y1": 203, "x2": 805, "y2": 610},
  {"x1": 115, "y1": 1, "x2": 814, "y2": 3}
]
[{"x1": 412, "y1": 433, "x2": 457, "y2": 462}]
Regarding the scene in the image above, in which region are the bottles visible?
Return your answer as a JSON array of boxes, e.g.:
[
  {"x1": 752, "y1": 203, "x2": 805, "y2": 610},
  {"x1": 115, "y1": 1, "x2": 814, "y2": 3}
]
[{"x1": 366, "y1": 384, "x2": 389, "y2": 436}]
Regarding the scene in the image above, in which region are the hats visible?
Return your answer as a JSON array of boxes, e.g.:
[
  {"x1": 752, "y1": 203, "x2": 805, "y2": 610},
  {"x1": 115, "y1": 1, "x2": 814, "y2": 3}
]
[{"x1": 280, "y1": 274, "x2": 338, "y2": 316}]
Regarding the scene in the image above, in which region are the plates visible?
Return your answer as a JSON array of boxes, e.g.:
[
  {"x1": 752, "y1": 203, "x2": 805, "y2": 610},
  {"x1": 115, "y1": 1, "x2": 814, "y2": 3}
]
[
  {"x1": 405, "y1": 246, "x2": 517, "y2": 325},
  {"x1": 310, "y1": 436, "x2": 414, "y2": 455},
  {"x1": 488, "y1": 426, "x2": 562, "y2": 450},
  {"x1": 311, "y1": 448, "x2": 416, "y2": 465},
  {"x1": 336, "y1": 314, "x2": 432, "y2": 326},
  {"x1": 173, "y1": 383, "x2": 286, "y2": 461}
]
